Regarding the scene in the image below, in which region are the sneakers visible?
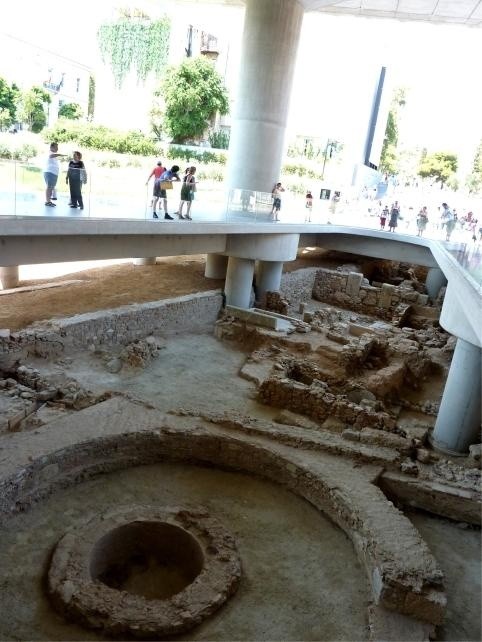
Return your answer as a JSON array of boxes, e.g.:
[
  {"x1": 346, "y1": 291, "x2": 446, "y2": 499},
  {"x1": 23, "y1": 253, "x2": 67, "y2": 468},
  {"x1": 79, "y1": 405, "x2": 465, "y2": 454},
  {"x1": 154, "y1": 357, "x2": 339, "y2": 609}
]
[
  {"x1": 153, "y1": 212, "x2": 158, "y2": 218},
  {"x1": 45, "y1": 202, "x2": 57, "y2": 208},
  {"x1": 165, "y1": 215, "x2": 173, "y2": 219}
]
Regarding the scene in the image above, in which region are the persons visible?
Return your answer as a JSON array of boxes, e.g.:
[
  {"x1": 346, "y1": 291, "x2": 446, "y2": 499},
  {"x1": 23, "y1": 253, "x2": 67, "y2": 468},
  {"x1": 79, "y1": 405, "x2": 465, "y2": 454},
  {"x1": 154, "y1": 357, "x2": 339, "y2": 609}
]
[
  {"x1": 355, "y1": 170, "x2": 482, "y2": 243},
  {"x1": 272, "y1": 180, "x2": 285, "y2": 222},
  {"x1": 144, "y1": 162, "x2": 166, "y2": 208},
  {"x1": 152, "y1": 166, "x2": 181, "y2": 221},
  {"x1": 43, "y1": 143, "x2": 68, "y2": 208},
  {"x1": 177, "y1": 167, "x2": 197, "y2": 221},
  {"x1": 303, "y1": 191, "x2": 315, "y2": 225},
  {"x1": 327, "y1": 188, "x2": 340, "y2": 225},
  {"x1": 239, "y1": 191, "x2": 255, "y2": 214},
  {"x1": 64, "y1": 151, "x2": 87, "y2": 211}
]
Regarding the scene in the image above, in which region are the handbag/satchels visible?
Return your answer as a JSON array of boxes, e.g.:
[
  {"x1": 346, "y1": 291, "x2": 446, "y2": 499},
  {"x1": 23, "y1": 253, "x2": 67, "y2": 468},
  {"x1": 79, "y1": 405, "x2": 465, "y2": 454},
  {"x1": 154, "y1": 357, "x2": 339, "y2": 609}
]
[
  {"x1": 79, "y1": 168, "x2": 85, "y2": 182},
  {"x1": 160, "y1": 181, "x2": 173, "y2": 190}
]
